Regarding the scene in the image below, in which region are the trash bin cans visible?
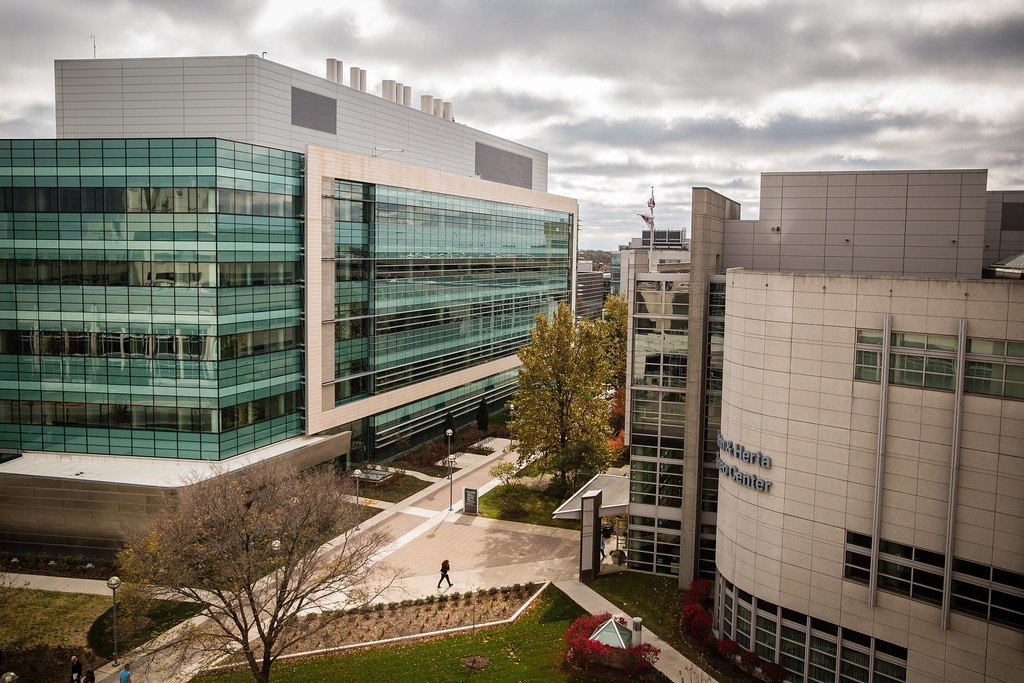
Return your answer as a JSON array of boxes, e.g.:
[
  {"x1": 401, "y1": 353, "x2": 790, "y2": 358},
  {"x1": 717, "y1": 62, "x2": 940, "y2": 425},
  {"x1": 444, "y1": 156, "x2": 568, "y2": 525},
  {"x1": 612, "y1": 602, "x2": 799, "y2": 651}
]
[{"x1": 601, "y1": 523, "x2": 613, "y2": 538}]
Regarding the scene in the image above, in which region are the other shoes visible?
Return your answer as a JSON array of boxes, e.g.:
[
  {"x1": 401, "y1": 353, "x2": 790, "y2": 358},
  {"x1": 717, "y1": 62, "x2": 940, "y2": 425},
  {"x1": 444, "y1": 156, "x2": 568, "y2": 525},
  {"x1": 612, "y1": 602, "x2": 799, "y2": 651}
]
[
  {"x1": 437, "y1": 586, "x2": 441, "y2": 588},
  {"x1": 449, "y1": 584, "x2": 453, "y2": 587}
]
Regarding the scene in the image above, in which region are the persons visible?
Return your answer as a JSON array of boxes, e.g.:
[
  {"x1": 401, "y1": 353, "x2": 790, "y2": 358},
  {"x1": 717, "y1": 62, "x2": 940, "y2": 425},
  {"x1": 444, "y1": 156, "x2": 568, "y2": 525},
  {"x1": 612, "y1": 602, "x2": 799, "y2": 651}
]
[
  {"x1": 70, "y1": 656, "x2": 82, "y2": 683},
  {"x1": 83, "y1": 670, "x2": 95, "y2": 683},
  {"x1": 437, "y1": 560, "x2": 453, "y2": 588},
  {"x1": 600, "y1": 539, "x2": 605, "y2": 558},
  {"x1": 120, "y1": 664, "x2": 131, "y2": 683}
]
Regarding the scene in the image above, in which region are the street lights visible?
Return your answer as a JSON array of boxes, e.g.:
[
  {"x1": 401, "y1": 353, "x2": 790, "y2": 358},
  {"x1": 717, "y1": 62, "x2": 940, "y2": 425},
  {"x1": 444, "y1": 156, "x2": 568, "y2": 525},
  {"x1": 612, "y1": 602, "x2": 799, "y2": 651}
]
[
  {"x1": 446, "y1": 429, "x2": 454, "y2": 511},
  {"x1": 107, "y1": 577, "x2": 121, "y2": 667},
  {"x1": 352, "y1": 469, "x2": 363, "y2": 531},
  {"x1": 510, "y1": 403, "x2": 515, "y2": 450},
  {"x1": 272, "y1": 540, "x2": 281, "y2": 606}
]
